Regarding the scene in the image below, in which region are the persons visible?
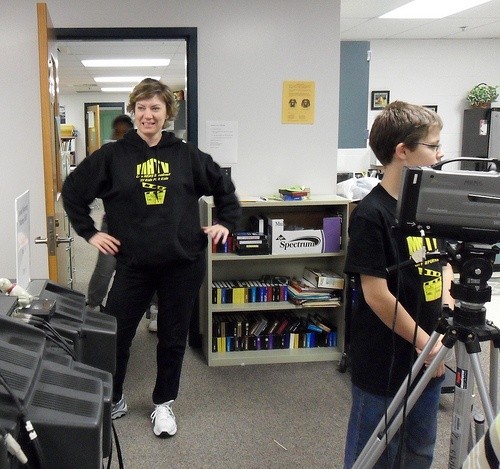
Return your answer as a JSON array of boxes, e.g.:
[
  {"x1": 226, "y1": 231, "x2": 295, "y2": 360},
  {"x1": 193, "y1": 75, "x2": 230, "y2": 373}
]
[
  {"x1": 62, "y1": 80, "x2": 243, "y2": 433},
  {"x1": 343, "y1": 100, "x2": 456, "y2": 469},
  {"x1": 85, "y1": 114, "x2": 159, "y2": 332}
]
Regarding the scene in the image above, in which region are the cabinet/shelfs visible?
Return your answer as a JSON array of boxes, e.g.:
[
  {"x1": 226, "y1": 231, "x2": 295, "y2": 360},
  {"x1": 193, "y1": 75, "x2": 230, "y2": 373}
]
[{"x1": 201, "y1": 194, "x2": 350, "y2": 367}]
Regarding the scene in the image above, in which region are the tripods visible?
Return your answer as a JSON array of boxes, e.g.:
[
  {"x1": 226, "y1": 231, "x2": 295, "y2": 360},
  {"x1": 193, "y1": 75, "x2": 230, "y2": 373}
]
[{"x1": 349, "y1": 312, "x2": 500, "y2": 469}]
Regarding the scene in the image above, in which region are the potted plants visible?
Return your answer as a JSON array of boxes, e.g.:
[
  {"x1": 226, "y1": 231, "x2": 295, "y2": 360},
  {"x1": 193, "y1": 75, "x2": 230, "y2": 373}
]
[{"x1": 465, "y1": 84, "x2": 500, "y2": 109}]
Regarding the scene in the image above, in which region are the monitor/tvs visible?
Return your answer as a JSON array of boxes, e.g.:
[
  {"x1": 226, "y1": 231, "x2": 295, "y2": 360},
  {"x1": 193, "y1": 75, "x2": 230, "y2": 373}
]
[
  {"x1": 26, "y1": 276, "x2": 118, "y2": 376},
  {"x1": 0, "y1": 314, "x2": 115, "y2": 469}
]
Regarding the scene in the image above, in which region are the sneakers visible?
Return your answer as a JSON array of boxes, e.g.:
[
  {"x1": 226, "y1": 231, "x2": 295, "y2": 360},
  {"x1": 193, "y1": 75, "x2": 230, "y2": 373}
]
[
  {"x1": 150, "y1": 400, "x2": 178, "y2": 437},
  {"x1": 111, "y1": 393, "x2": 128, "y2": 420}
]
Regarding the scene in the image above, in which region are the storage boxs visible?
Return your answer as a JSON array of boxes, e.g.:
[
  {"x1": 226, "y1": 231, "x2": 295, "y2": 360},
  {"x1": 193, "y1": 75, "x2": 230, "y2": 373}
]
[
  {"x1": 303, "y1": 267, "x2": 345, "y2": 290},
  {"x1": 264, "y1": 213, "x2": 341, "y2": 256}
]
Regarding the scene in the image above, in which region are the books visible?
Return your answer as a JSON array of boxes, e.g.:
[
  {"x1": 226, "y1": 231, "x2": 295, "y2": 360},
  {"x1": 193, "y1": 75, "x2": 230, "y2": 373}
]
[
  {"x1": 60, "y1": 138, "x2": 75, "y2": 165},
  {"x1": 287, "y1": 276, "x2": 341, "y2": 311}
]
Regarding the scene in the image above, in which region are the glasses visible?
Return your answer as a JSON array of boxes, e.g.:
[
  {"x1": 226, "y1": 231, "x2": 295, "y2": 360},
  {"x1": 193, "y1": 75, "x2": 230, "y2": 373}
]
[{"x1": 394, "y1": 138, "x2": 443, "y2": 153}]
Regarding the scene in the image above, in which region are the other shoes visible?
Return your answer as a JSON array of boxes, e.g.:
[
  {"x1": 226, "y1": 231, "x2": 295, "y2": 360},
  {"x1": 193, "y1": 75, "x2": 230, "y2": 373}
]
[
  {"x1": 85, "y1": 304, "x2": 100, "y2": 313},
  {"x1": 147, "y1": 305, "x2": 159, "y2": 331}
]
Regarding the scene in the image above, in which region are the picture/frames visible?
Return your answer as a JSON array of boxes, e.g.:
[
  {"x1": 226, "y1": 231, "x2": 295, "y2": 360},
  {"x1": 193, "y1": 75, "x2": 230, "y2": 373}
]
[
  {"x1": 371, "y1": 91, "x2": 390, "y2": 110},
  {"x1": 421, "y1": 105, "x2": 437, "y2": 113}
]
